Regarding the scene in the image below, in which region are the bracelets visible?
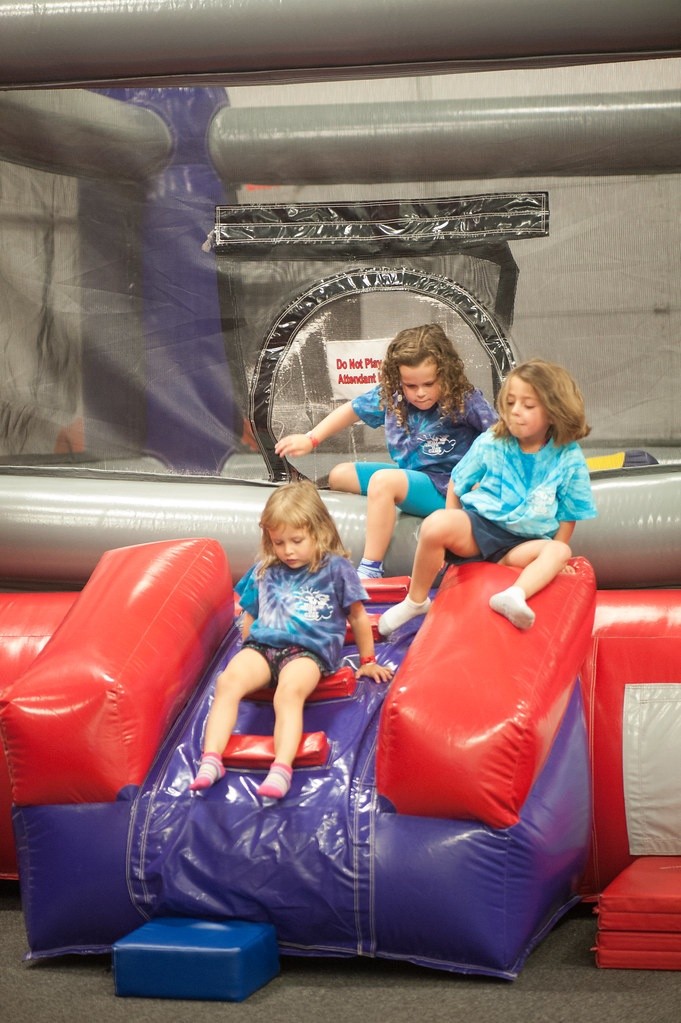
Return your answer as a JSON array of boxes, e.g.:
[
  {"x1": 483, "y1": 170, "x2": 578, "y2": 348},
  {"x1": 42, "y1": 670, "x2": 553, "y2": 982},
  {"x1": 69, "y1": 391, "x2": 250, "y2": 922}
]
[
  {"x1": 305, "y1": 430, "x2": 319, "y2": 447},
  {"x1": 359, "y1": 656, "x2": 376, "y2": 665}
]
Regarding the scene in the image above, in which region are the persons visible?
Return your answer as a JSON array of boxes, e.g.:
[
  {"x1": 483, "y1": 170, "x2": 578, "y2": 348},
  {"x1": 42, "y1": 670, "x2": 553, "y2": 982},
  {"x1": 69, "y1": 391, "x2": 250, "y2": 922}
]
[
  {"x1": 377, "y1": 358, "x2": 598, "y2": 635},
  {"x1": 188, "y1": 481, "x2": 395, "y2": 798},
  {"x1": 275, "y1": 323, "x2": 500, "y2": 579}
]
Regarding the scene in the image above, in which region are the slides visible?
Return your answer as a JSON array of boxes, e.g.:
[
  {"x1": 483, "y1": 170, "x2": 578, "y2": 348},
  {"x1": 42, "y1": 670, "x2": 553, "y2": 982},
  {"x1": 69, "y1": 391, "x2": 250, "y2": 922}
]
[{"x1": 0, "y1": 537, "x2": 611, "y2": 966}]
